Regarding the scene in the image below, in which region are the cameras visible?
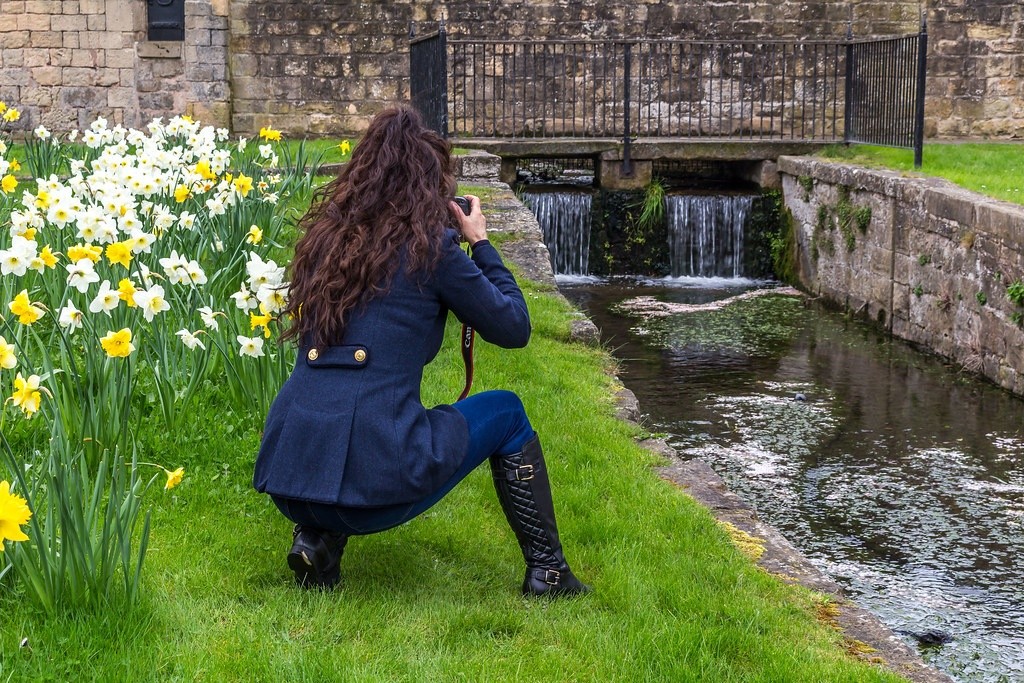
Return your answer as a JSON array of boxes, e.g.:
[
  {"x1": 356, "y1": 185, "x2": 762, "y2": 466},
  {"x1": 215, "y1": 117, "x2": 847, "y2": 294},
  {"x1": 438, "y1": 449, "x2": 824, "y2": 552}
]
[{"x1": 447, "y1": 196, "x2": 470, "y2": 243}]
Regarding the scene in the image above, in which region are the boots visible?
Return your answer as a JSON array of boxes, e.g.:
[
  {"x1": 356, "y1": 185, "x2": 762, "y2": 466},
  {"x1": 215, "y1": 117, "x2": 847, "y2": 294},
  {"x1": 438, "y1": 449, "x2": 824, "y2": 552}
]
[
  {"x1": 286, "y1": 525, "x2": 348, "y2": 589},
  {"x1": 488, "y1": 430, "x2": 589, "y2": 597}
]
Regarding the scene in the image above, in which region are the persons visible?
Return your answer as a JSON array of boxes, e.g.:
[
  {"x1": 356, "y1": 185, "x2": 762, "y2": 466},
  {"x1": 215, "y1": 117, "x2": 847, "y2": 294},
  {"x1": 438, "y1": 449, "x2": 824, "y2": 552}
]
[{"x1": 253, "y1": 108, "x2": 591, "y2": 598}]
